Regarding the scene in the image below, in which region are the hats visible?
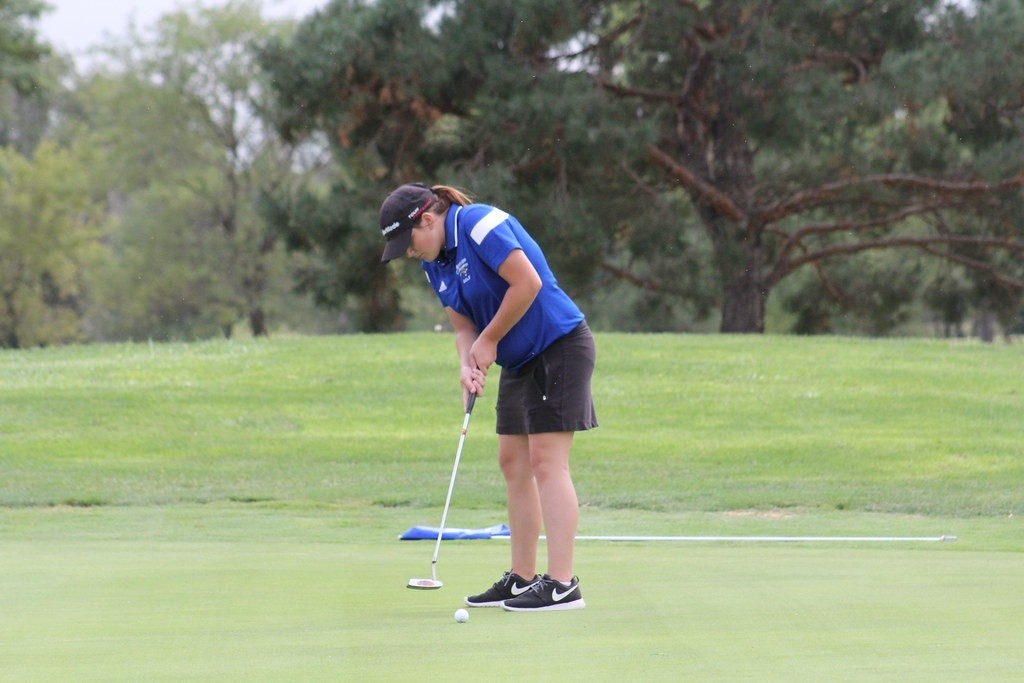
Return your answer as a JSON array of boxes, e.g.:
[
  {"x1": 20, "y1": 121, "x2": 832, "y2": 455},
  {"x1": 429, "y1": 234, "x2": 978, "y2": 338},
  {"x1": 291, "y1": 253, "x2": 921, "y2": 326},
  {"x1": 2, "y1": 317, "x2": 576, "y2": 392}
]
[{"x1": 378, "y1": 184, "x2": 436, "y2": 262}]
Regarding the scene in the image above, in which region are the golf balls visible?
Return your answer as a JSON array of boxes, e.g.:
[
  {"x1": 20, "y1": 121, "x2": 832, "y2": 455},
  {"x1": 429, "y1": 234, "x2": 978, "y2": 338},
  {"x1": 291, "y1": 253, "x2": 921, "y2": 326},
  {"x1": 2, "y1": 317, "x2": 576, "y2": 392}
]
[{"x1": 455, "y1": 608, "x2": 468, "y2": 622}]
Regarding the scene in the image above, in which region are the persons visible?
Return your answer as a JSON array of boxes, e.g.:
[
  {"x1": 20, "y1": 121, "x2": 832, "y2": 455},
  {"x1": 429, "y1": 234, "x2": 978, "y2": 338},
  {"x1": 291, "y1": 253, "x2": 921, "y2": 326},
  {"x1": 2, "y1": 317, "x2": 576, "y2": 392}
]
[{"x1": 380, "y1": 181, "x2": 599, "y2": 614}]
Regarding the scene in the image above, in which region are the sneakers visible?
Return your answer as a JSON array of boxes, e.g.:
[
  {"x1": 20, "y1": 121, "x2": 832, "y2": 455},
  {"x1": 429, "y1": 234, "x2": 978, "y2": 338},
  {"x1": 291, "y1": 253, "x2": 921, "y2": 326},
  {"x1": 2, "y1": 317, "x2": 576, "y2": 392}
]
[
  {"x1": 465, "y1": 573, "x2": 542, "y2": 606},
  {"x1": 502, "y1": 575, "x2": 586, "y2": 612}
]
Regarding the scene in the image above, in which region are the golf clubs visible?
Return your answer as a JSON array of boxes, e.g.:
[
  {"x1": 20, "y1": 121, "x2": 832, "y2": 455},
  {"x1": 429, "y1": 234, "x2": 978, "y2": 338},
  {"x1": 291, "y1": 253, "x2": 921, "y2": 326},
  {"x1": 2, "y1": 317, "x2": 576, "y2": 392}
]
[{"x1": 406, "y1": 394, "x2": 478, "y2": 589}]
